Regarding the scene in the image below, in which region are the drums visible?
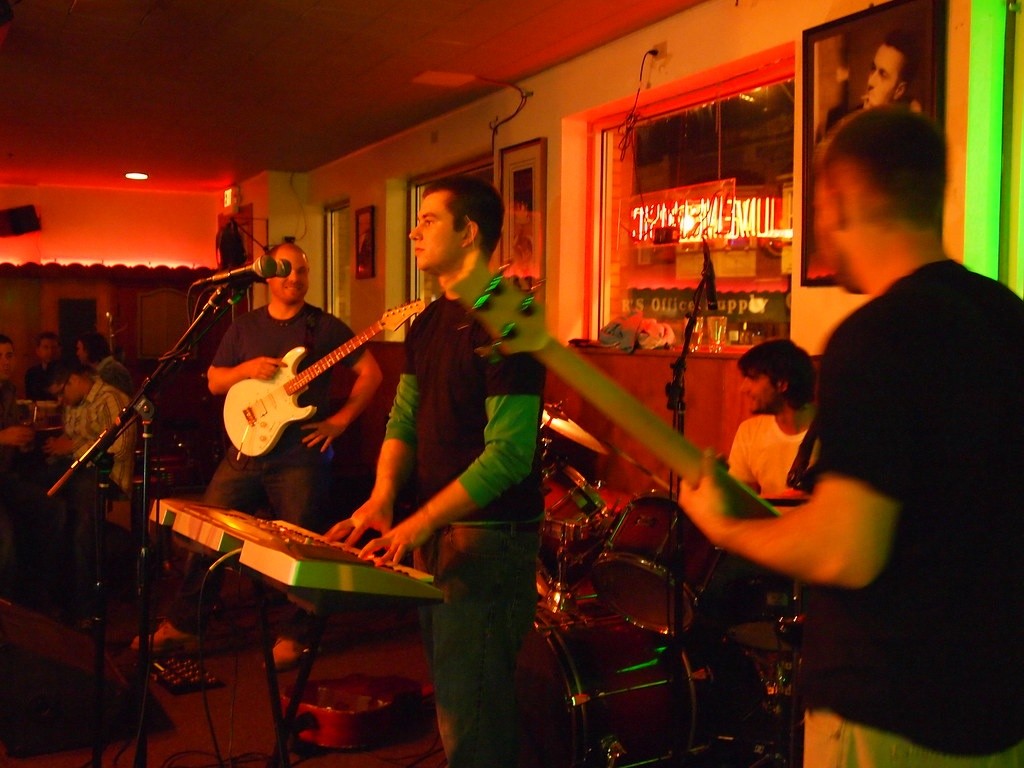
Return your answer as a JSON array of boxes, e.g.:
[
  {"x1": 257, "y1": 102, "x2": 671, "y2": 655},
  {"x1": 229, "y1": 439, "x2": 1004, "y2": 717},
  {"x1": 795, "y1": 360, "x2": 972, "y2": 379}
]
[
  {"x1": 514, "y1": 599, "x2": 697, "y2": 767},
  {"x1": 534, "y1": 447, "x2": 615, "y2": 599},
  {"x1": 588, "y1": 487, "x2": 726, "y2": 637}
]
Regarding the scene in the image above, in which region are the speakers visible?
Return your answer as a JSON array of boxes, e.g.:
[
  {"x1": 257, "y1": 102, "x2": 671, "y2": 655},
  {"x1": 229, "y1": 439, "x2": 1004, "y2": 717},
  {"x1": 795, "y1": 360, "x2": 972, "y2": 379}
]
[{"x1": 0, "y1": 598, "x2": 175, "y2": 759}]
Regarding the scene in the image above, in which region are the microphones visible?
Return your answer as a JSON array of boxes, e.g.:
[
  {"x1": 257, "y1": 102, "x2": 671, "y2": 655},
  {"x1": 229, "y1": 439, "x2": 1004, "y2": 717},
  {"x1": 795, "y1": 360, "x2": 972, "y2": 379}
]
[
  {"x1": 192, "y1": 254, "x2": 291, "y2": 294},
  {"x1": 704, "y1": 246, "x2": 719, "y2": 310}
]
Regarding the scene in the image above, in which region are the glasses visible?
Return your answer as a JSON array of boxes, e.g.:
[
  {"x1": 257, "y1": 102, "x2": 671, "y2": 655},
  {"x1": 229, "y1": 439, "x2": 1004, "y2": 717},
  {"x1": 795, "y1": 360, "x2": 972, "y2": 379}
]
[{"x1": 53, "y1": 372, "x2": 76, "y2": 397}]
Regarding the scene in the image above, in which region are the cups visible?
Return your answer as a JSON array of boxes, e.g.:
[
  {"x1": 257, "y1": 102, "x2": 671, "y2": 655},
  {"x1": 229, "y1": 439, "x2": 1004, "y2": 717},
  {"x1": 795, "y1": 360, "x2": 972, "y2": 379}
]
[{"x1": 683, "y1": 317, "x2": 728, "y2": 353}]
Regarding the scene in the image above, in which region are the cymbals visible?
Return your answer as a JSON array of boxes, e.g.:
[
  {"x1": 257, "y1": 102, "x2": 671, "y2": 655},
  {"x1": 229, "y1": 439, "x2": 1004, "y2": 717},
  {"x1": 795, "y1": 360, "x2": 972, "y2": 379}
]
[
  {"x1": 761, "y1": 489, "x2": 809, "y2": 505},
  {"x1": 538, "y1": 403, "x2": 610, "y2": 457}
]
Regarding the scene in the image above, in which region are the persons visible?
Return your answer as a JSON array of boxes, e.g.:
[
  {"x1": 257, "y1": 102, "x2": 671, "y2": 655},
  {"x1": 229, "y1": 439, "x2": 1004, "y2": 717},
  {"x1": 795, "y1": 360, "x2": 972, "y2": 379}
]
[
  {"x1": 863, "y1": 30, "x2": 932, "y2": 110},
  {"x1": 691, "y1": 339, "x2": 820, "y2": 714},
  {"x1": 127, "y1": 243, "x2": 381, "y2": 670},
  {"x1": 325, "y1": 176, "x2": 548, "y2": 768},
  {"x1": 0, "y1": 331, "x2": 136, "y2": 626},
  {"x1": 678, "y1": 109, "x2": 1024, "y2": 768}
]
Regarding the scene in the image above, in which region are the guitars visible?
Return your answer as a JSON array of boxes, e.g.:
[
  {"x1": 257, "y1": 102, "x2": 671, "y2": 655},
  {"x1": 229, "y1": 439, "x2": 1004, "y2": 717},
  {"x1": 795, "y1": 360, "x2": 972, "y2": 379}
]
[
  {"x1": 441, "y1": 246, "x2": 784, "y2": 526},
  {"x1": 223, "y1": 295, "x2": 428, "y2": 459}
]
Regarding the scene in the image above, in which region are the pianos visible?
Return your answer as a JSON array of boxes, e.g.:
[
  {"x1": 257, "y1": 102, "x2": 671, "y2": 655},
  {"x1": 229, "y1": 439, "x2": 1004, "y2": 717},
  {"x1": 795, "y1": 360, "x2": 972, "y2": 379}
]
[{"x1": 148, "y1": 494, "x2": 449, "y2": 767}]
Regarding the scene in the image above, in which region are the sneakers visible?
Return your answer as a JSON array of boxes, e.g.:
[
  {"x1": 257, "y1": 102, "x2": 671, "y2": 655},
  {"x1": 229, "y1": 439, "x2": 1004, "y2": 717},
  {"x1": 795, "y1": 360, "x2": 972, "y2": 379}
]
[
  {"x1": 272, "y1": 634, "x2": 306, "y2": 669},
  {"x1": 131, "y1": 616, "x2": 198, "y2": 656}
]
